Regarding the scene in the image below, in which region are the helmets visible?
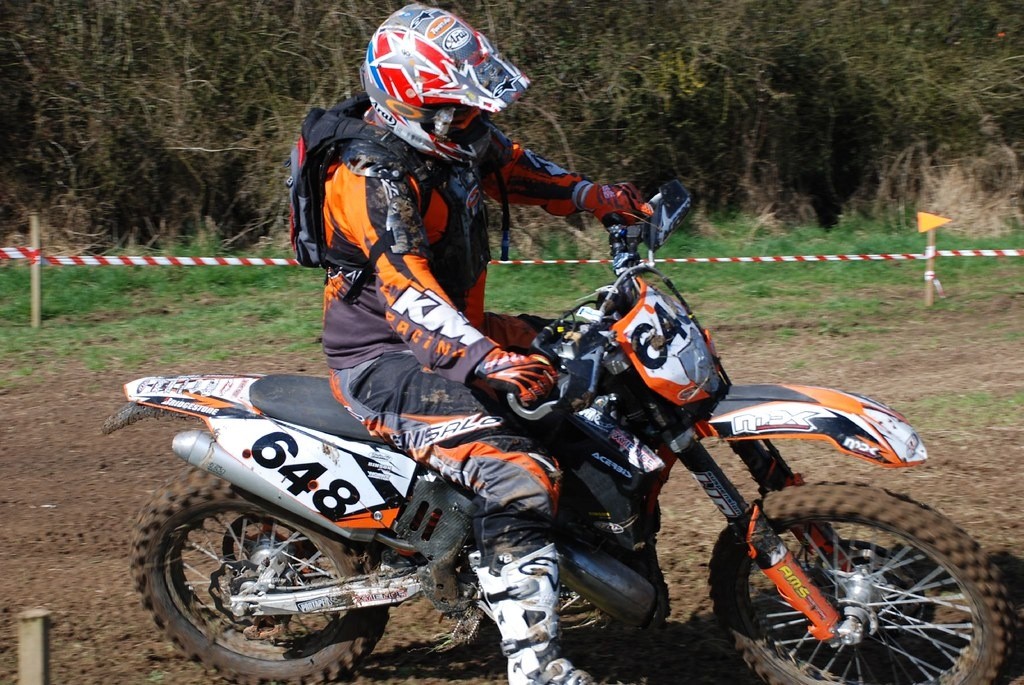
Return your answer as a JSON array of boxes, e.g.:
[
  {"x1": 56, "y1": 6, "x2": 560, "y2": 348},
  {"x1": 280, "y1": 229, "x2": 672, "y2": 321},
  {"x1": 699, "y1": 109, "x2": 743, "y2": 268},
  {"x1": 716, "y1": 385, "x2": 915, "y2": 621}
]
[{"x1": 364, "y1": 4, "x2": 527, "y2": 164}]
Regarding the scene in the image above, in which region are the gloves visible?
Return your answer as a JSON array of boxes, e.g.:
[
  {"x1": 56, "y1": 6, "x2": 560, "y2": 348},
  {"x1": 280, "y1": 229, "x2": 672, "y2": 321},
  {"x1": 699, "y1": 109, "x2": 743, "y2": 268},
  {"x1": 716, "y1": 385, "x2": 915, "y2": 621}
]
[
  {"x1": 583, "y1": 182, "x2": 653, "y2": 231},
  {"x1": 474, "y1": 348, "x2": 561, "y2": 409}
]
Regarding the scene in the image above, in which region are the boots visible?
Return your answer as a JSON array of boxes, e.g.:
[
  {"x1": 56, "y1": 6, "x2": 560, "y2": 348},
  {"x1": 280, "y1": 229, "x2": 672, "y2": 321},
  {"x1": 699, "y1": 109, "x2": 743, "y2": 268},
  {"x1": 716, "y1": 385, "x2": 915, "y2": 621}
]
[{"x1": 467, "y1": 542, "x2": 593, "y2": 685}]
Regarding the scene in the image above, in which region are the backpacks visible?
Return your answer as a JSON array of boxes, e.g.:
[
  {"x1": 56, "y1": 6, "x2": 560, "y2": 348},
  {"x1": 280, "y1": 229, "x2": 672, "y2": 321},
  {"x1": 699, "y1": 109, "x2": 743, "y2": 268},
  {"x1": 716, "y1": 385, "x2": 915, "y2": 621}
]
[{"x1": 288, "y1": 101, "x2": 432, "y2": 278}]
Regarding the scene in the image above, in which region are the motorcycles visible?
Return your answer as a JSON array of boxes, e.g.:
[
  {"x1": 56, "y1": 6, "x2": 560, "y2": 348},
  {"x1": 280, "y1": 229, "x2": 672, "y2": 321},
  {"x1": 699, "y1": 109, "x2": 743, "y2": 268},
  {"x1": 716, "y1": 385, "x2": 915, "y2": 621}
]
[{"x1": 99, "y1": 178, "x2": 1017, "y2": 685}]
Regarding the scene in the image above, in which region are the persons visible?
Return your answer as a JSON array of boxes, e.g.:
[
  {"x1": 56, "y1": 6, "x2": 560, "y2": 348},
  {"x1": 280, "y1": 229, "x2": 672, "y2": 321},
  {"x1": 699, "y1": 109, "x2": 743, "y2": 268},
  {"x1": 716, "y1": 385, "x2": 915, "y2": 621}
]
[{"x1": 323, "y1": 2, "x2": 653, "y2": 685}]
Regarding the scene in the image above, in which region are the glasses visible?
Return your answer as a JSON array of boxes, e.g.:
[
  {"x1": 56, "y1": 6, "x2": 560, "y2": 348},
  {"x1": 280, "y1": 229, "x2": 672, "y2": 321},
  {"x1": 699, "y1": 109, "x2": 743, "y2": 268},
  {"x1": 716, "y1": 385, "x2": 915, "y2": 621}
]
[{"x1": 361, "y1": 64, "x2": 483, "y2": 130}]
